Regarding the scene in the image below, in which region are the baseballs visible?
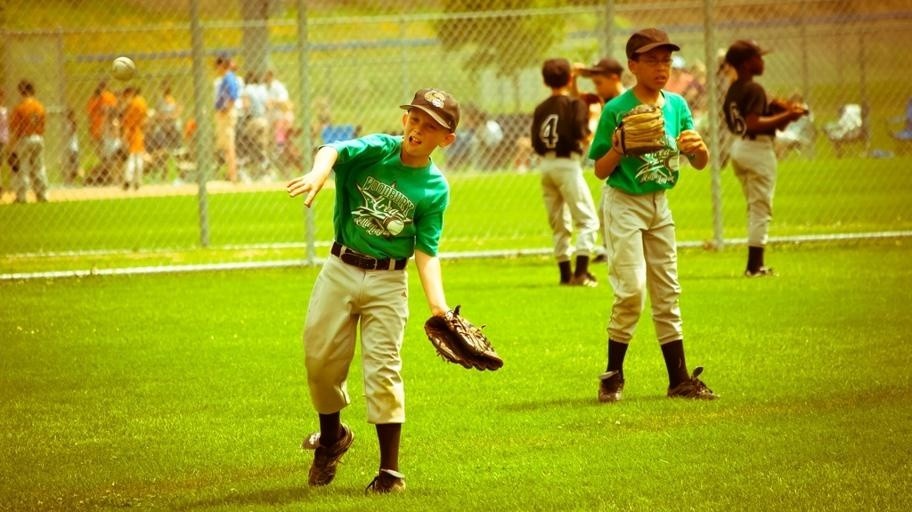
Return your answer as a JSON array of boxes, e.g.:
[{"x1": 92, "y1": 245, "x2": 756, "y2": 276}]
[{"x1": 112, "y1": 57, "x2": 136, "y2": 79}]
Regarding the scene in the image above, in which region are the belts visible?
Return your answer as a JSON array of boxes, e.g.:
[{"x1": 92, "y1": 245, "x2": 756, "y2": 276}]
[
  {"x1": 330, "y1": 242, "x2": 406, "y2": 271},
  {"x1": 741, "y1": 133, "x2": 757, "y2": 141},
  {"x1": 541, "y1": 150, "x2": 571, "y2": 158}
]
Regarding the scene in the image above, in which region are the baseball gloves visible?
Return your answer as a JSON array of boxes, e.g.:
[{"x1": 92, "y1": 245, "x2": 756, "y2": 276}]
[
  {"x1": 768, "y1": 98, "x2": 807, "y2": 132},
  {"x1": 424, "y1": 313, "x2": 502, "y2": 371},
  {"x1": 612, "y1": 103, "x2": 666, "y2": 156}
]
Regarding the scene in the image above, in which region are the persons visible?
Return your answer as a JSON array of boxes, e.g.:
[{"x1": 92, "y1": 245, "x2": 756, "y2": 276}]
[
  {"x1": 447, "y1": 110, "x2": 541, "y2": 173},
  {"x1": 86, "y1": 81, "x2": 194, "y2": 191},
  {"x1": 211, "y1": 57, "x2": 330, "y2": 185},
  {"x1": 828, "y1": 106, "x2": 863, "y2": 157},
  {"x1": 287, "y1": 88, "x2": 459, "y2": 495},
  {"x1": 589, "y1": 30, "x2": 719, "y2": 403},
  {"x1": 62, "y1": 110, "x2": 80, "y2": 181},
  {"x1": 1, "y1": 80, "x2": 48, "y2": 203},
  {"x1": 725, "y1": 41, "x2": 804, "y2": 277},
  {"x1": 776, "y1": 95, "x2": 815, "y2": 160},
  {"x1": 531, "y1": 59, "x2": 599, "y2": 286},
  {"x1": 568, "y1": 58, "x2": 627, "y2": 263}
]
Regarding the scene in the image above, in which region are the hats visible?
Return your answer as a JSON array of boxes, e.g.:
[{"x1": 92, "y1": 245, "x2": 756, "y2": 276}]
[
  {"x1": 581, "y1": 61, "x2": 622, "y2": 78},
  {"x1": 400, "y1": 89, "x2": 459, "y2": 133},
  {"x1": 724, "y1": 40, "x2": 770, "y2": 67},
  {"x1": 543, "y1": 59, "x2": 570, "y2": 87},
  {"x1": 626, "y1": 29, "x2": 680, "y2": 58}
]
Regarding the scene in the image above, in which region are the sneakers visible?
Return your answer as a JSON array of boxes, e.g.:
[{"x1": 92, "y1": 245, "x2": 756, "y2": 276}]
[
  {"x1": 366, "y1": 470, "x2": 403, "y2": 493},
  {"x1": 598, "y1": 370, "x2": 624, "y2": 403},
  {"x1": 303, "y1": 425, "x2": 356, "y2": 488},
  {"x1": 743, "y1": 268, "x2": 775, "y2": 278},
  {"x1": 668, "y1": 368, "x2": 718, "y2": 400},
  {"x1": 562, "y1": 273, "x2": 597, "y2": 288}
]
